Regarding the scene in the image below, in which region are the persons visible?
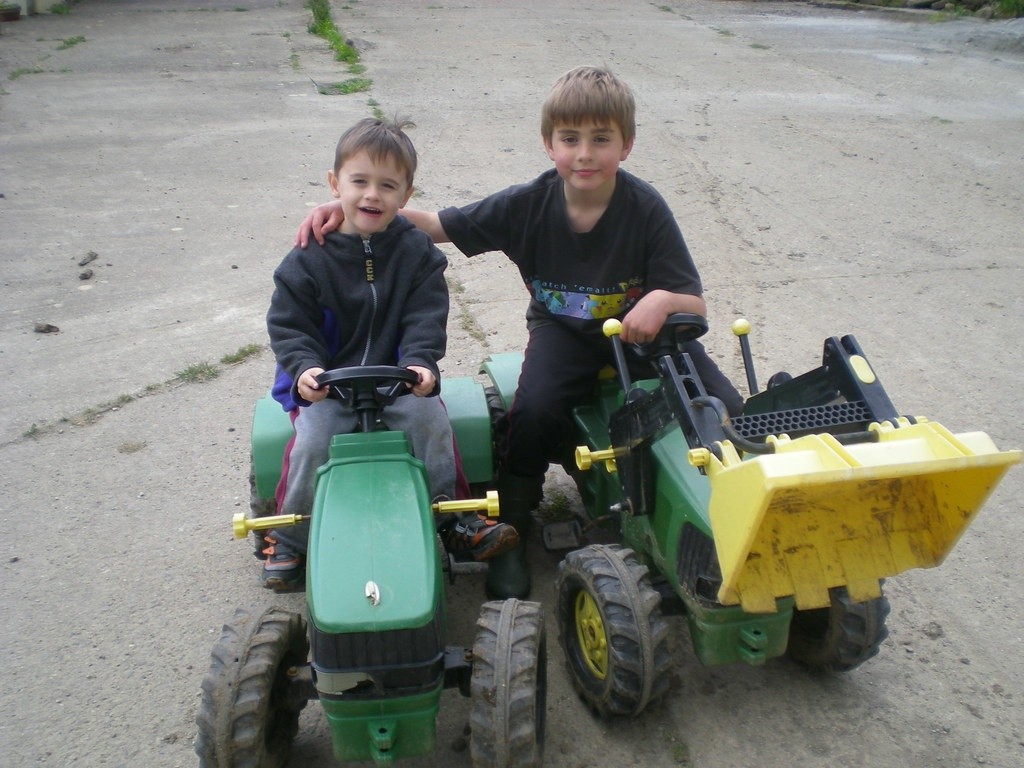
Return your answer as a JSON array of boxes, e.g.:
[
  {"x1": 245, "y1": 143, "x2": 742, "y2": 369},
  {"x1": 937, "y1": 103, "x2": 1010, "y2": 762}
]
[
  {"x1": 294, "y1": 64, "x2": 748, "y2": 598},
  {"x1": 259, "y1": 114, "x2": 519, "y2": 589}
]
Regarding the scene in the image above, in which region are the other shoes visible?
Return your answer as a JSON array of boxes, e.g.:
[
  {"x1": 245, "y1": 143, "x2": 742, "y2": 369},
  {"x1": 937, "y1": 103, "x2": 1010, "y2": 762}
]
[
  {"x1": 766, "y1": 371, "x2": 793, "y2": 389},
  {"x1": 485, "y1": 518, "x2": 531, "y2": 599}
]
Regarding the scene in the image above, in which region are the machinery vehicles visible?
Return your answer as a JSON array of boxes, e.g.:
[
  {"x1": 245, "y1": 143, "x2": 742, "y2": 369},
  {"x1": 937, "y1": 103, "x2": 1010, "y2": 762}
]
[{"x1": 478, "y1": 303, "x2": 1024, "y2": 723}]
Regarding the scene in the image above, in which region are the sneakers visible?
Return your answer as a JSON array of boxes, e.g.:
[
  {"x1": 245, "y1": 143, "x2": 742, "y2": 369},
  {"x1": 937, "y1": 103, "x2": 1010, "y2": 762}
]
[
  {"x1": 263, "y1": 533, "x2": 302, "y2": 589},
  {"x1": 440, "y1": 511, "x2": 518, "y2": 561}
]
